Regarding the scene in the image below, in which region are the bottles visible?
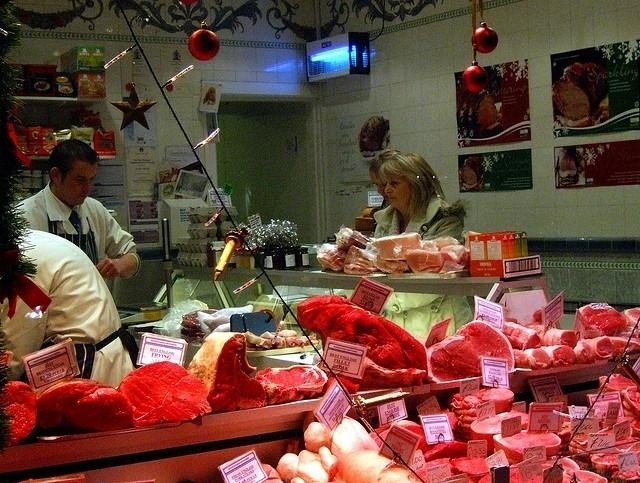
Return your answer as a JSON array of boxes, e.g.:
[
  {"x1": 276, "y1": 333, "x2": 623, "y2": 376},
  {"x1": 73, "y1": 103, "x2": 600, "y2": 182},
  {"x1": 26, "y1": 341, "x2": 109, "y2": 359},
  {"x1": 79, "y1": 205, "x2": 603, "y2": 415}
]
[{"x1": 254, "y1": 246, "x2": 312, "y2": 270}]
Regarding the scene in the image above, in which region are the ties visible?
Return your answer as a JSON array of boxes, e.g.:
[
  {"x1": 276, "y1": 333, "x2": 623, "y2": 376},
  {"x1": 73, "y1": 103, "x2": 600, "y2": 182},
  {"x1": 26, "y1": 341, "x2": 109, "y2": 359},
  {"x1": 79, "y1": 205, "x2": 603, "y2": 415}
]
[{"x1": 69, "y1": 211, "x2": 82, "y2": 234}]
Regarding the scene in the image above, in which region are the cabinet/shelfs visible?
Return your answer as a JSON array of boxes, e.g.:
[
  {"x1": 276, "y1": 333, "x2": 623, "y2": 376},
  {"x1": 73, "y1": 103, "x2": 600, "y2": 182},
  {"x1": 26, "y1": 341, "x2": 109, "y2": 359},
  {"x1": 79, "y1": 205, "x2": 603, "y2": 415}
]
[
  {"x1": 12, "y1": 95, "x2": 116, "y2": 161},
  {"x1": 108, "y1": 260, "x2": 561, "y2": 351}
]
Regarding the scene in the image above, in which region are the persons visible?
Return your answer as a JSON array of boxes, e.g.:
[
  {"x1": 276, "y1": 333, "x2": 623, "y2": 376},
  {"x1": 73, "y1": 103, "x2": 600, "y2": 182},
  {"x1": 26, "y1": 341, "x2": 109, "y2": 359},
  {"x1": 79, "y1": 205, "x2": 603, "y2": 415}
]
[
  {"x1": 368, "y1": 149, "x2": 407, "y2": 233},
  {"x1": 374, "y1": 152, "x2": 472, "y2": 350},
  {"x1": 13, "y1": 137, "x2": 141, "y2": 291},
  {"x1": 2, "y1": 228, "x2": 137, "y2": 390}
]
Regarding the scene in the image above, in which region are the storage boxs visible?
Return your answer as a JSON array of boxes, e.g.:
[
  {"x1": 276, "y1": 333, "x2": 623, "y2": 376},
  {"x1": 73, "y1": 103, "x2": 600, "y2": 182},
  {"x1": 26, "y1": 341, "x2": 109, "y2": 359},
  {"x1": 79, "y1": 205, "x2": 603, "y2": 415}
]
[
  {"x1": 61, "y1": 45, "x2": 105, "y2": 73},
  {"x1": 70, "y1": 71, "x2": 106, "y2": 99}
]
